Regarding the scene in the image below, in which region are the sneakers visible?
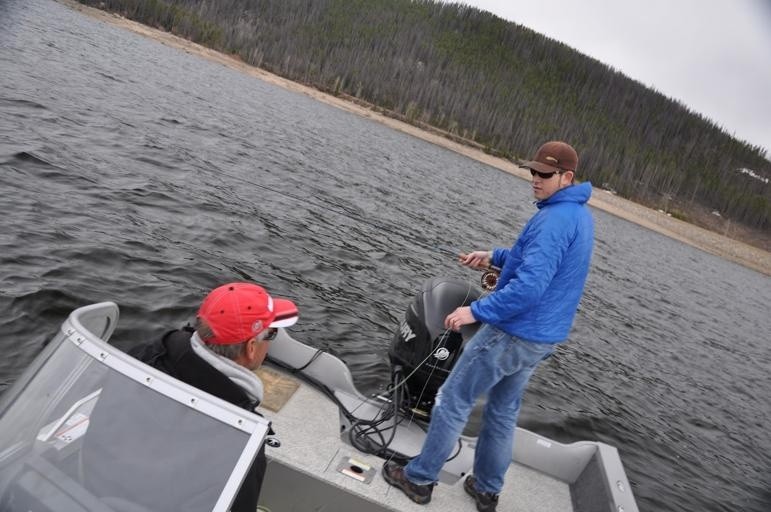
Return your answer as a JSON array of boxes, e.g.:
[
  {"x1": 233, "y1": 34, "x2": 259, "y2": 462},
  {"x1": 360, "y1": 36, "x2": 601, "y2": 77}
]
[
  {"x1": 465, "y1": 476, "x2": 499, "y2": 512},
  {"x1": 384, "y1": 463, "x2": 438, "y2": 504}
]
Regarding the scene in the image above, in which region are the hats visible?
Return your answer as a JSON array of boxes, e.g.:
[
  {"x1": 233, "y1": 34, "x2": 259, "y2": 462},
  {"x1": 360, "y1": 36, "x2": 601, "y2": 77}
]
[
  {"x1": 519, "y1": 140, "x2": 578, "y2": 173},
  {"x1": 195, "y1": 282, "x2": 299, "y2": 344}
]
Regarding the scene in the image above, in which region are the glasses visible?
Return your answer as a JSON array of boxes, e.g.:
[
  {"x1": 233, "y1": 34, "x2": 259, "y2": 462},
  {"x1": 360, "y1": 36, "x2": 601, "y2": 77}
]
[
  {"x1": 263, "y1": 328, "x2": 277, "y2": 340},
  {"x1": 530, "y1": 168, "x2": 561, "y2": 178}
]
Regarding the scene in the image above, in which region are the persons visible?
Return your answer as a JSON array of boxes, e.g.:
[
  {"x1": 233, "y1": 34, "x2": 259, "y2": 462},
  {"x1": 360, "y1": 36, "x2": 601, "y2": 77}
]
[
  {"x1": 382, "y1": 141, "x2": 596, "y2": 512},
  {"x1": 55, "y1": 281, "x2": 299, "y2": 512}
]
